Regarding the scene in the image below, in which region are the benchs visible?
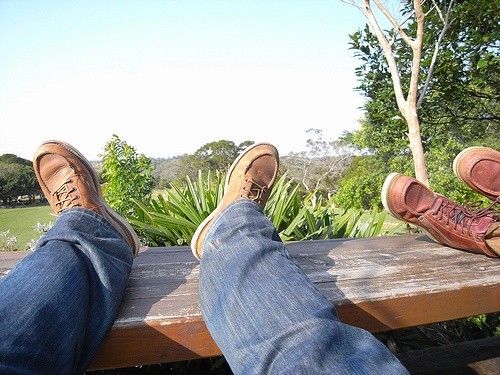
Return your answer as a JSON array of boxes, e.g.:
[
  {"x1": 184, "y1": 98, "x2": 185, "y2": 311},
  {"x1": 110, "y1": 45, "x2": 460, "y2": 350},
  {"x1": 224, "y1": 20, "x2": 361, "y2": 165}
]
[{"x1": 0, "y1": 232, "x2": 500, "y2": 370}]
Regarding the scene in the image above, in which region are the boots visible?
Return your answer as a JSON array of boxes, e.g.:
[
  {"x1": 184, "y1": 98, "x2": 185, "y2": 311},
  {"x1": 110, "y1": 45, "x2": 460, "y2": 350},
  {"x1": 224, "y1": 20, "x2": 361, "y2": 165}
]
[
  {"x1": 380, "y1": 170, "x2": 500, "y2": 259},
  {"x1": 452, "y1": 146, "x2": 500, "y2": 203}
]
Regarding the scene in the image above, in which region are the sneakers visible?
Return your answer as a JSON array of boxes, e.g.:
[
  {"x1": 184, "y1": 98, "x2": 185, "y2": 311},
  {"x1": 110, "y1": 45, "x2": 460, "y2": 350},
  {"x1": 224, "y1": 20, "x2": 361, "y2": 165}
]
[
  {"x1": 190, "y1": 141, "x2": 280, "y2": 262},
  {"x1": 32, "y1": 139, "x2": 140, "y2": 260}
]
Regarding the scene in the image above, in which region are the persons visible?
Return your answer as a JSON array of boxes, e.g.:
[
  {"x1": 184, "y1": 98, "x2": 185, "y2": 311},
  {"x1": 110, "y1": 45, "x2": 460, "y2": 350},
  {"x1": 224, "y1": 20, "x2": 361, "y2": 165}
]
[
  {"x1": 0, "y1": 143, "x2": 410, "y2": 375},
  {"x1": 382, "y1": 146, "x2": 500, "y2": 256}
]
